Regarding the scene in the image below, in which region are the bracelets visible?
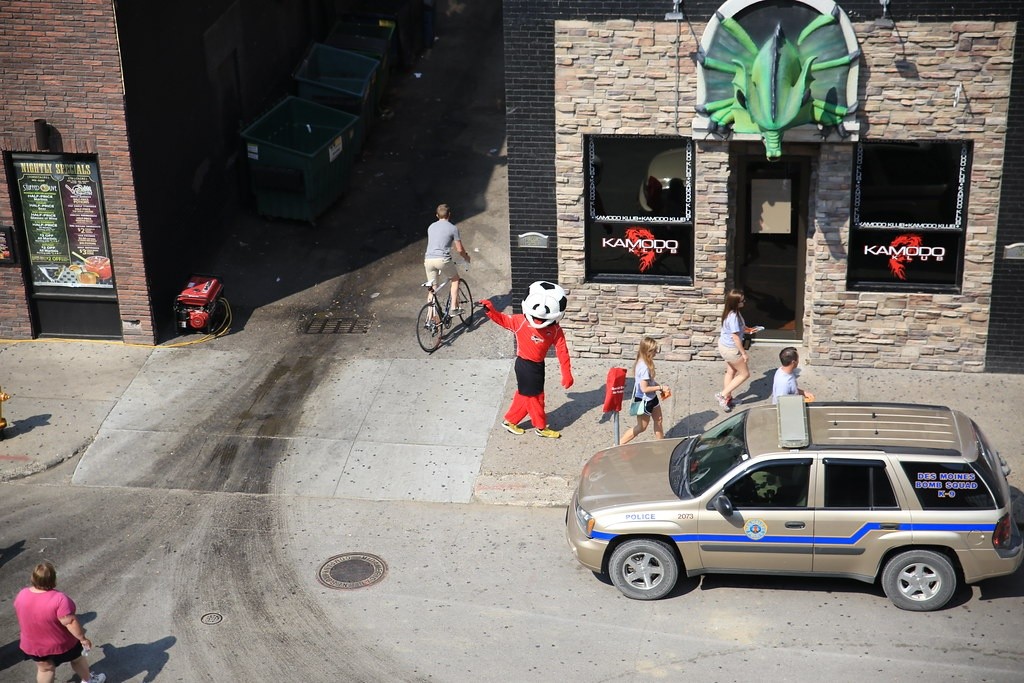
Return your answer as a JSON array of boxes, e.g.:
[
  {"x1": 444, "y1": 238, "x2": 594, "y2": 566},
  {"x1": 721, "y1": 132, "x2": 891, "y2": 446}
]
[
  {"x1": 659, "y1": 385, "x2": 663, "y2": 391},
  {"x1": 742, "y1": 352, "x2": 746, "y2": 355}
]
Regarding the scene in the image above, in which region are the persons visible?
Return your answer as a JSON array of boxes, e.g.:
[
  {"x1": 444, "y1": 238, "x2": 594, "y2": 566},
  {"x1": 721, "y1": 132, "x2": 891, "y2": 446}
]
[
  {"x1": 715, "y1": 288, "x2": 758, "y2": 412},
  {"x1": 770, "y1": 347, "x2": 816, "y2": 405},
  {"x1": 424, "y1": 203, "x2": 471, "y2": 327},
  {"x1": 13, "y1": 562, "x2": 107, "y2": 683},
  {"x1": 613, "y1": 337, "x2": 672, "y2": 446}
]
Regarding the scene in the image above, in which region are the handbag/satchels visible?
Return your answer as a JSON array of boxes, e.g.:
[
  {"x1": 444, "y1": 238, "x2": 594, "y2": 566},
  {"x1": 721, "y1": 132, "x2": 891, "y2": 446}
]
[{"x1": 629, "y1": 399, "x2": 647, "y2": 416}]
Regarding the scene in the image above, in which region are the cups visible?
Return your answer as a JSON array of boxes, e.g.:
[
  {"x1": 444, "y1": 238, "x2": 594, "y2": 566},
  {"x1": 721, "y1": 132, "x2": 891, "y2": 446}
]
[
  {"x1": 84, "y1": 256, "x2": 112, "y2": 280},
  {"x1": 78, "y1": 273, "x2": 96, "y2": 284}
]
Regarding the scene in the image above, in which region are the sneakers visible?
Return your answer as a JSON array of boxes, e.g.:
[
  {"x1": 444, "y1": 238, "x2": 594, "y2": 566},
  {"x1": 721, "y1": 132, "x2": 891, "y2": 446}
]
[
  {"x1": 450, "y1": 307, "x2": 466, "y2": 317},
  {"x1": 424, "y1": 322, "x2": 431, "y2": 330},
  {"x1": 81, "y1": 671, "x2": 106, "y2": 683},
  {"x1": 501, "y1": 419, "x2": 525, "y2": 435},
  {"x1": 535, "y1": 423, "x2": 560, "y2": 438}
]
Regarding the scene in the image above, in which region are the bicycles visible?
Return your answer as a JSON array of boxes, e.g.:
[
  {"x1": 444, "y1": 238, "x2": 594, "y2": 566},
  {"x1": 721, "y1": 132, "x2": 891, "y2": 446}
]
[{"x1": 415, "y1": 256, "x2": 474, "y2": 353}]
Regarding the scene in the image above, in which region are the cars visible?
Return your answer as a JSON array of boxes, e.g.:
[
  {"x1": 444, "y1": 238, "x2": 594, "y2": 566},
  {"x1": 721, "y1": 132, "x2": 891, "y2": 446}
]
[{"x1": 564, "y1": 394, "x2": 1024, "y2": 611}]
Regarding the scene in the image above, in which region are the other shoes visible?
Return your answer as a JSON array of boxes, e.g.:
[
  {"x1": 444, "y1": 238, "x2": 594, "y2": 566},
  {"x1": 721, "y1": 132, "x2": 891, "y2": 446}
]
[
  {"x1": 715, "y1": 392, "x2": 730, "y2": 412},
  {"x1": 727, "y1": 397, "x2": 742, "y2": 406}
]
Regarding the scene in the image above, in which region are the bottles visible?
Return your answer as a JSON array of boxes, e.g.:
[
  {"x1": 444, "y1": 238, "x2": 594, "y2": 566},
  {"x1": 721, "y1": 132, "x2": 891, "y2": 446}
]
[
  {"x1": 81, "y1": 646, "x2": 89, "y2": 657},
  {"x1": 747, "y1": 326, "x2": 766, "y2": 335}
]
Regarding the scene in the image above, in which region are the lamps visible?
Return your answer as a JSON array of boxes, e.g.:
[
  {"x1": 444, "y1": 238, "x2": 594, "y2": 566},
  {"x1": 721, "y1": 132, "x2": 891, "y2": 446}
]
[
  {"x1": 874, "y1": 0, "x2": 894, "y2": 27},
  {"x1": 665, "y1": 1, "x2": 684, "y2": 21}
]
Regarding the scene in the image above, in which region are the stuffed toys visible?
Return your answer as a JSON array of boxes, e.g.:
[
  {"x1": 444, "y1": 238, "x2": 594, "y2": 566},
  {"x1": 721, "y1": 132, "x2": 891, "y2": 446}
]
[{"x1": 480, "y1": 280, "x2": 574, "y2": 439}]
